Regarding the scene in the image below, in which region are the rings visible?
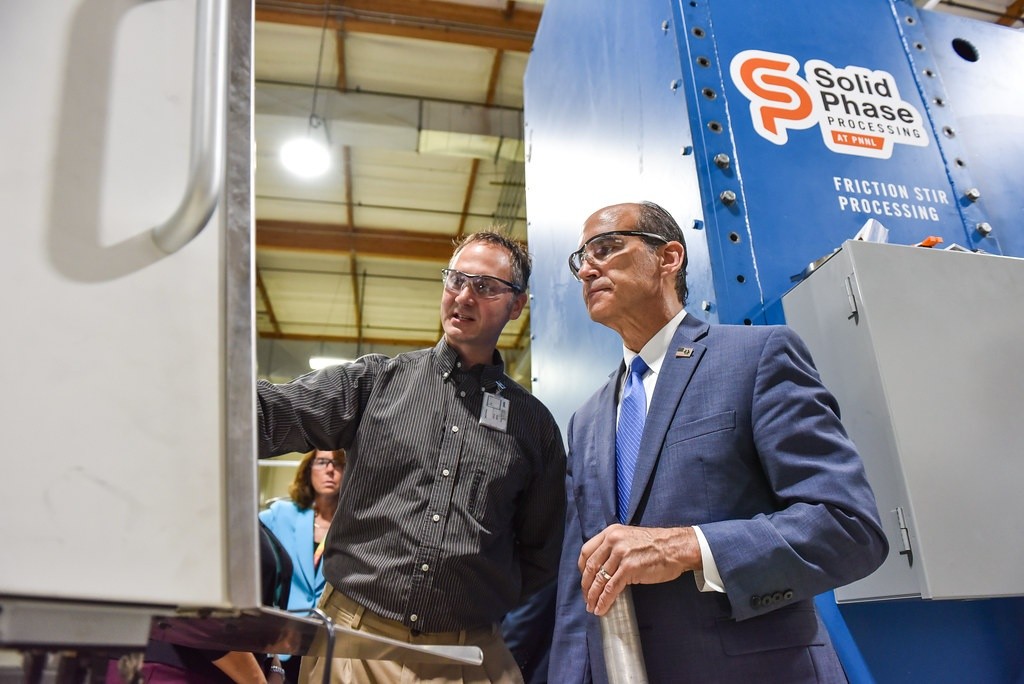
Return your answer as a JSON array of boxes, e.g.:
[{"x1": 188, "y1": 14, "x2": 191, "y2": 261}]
[{"x1": 598, "y1": 568, "x2": 612, "y2": 581}]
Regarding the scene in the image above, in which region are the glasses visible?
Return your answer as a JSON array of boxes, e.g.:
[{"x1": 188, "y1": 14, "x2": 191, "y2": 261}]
[
  {"x1": 310, "y1": 458, "x2": 345, "y2": 470},
  {"x1": 568, "y1": 231, "x2": 669, "y2": 282},
  {"x1": 440, "y1": 268, "x2": 522, "y2": 299}
]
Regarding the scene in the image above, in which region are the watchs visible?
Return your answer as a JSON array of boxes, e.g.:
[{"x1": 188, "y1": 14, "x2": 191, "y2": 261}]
[{"x1": 268, "y1": 665, "x2": 287, "y2": 682}]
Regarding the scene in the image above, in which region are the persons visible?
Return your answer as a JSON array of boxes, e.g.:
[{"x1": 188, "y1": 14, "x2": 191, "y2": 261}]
[
  {"x1": 104, "y1": 517, "x2": 294, "y2": 684},
  {"x1": 257, "y1": 225, "x2": 568, "y2": 684},
  {"x1": 257, "y1": 449, "x2": 346, "y2": 684},
  {"x1": 545, "y1": 200, "x2": 890, "y2": 684}
]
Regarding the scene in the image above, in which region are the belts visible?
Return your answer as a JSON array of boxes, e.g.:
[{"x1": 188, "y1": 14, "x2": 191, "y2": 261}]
[{"x1": 330, "y1": 594, "x2": 496, "y2": 645}]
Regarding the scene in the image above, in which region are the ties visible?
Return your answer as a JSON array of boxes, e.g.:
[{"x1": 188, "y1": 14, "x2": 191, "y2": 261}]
[{"x1": 615, "y1": 359, "x2": 650, "y2": 527}]
